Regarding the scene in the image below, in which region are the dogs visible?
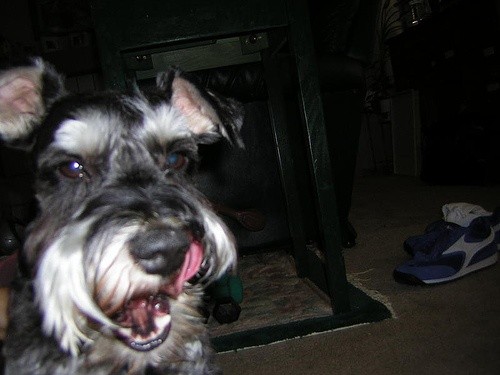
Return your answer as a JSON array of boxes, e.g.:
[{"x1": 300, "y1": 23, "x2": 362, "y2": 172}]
[{"x1": 1, "y1": 55, "x2": 245, "y2": 374}]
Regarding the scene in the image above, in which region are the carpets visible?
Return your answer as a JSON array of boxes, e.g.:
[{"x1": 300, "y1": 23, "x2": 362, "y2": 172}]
[{"x1": 206, "y1": 251, "x2": 396, "y2": 355}]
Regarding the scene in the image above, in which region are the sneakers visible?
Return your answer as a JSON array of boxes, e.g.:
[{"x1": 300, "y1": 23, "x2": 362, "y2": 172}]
[{"x1": 392, "y1": 203, "x2": 500, "y2": 286}]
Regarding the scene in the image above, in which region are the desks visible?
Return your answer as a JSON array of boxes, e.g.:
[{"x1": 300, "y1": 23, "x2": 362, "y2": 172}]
[{"x1": 90, "y1": 0, "x2": 352, "y2": 315}]
